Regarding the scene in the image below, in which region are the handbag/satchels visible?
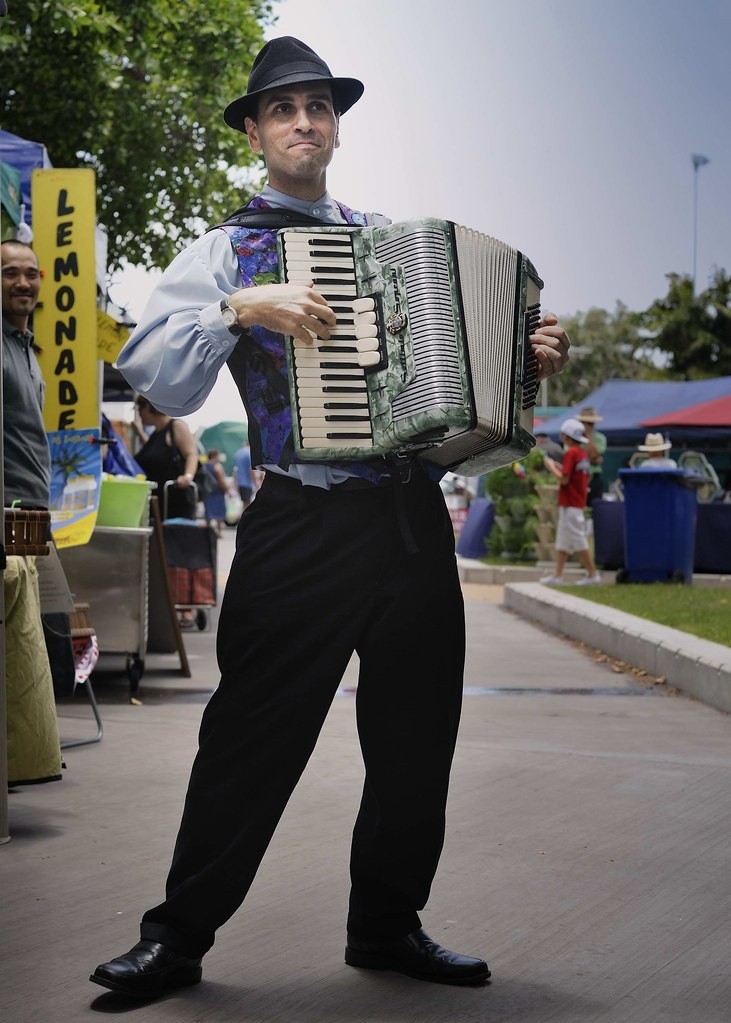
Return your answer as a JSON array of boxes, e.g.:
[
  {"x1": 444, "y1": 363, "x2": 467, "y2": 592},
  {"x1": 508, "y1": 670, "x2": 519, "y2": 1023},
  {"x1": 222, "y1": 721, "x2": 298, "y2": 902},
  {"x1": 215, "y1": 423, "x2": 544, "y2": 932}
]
[{"x1": 167, "y1": 418, "x2": 220, "y2": 502}]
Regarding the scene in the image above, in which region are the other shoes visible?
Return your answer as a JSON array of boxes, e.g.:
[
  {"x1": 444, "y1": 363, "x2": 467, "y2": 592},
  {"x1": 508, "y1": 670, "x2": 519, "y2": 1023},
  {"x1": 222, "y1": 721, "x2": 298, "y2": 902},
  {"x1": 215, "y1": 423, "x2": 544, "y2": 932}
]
[
  {"x1": 540, "y1": 576, "x2": 564, "y2": 584},
  {"x1": 576, "y1": 575, "x2": 600, "y2": 585}
]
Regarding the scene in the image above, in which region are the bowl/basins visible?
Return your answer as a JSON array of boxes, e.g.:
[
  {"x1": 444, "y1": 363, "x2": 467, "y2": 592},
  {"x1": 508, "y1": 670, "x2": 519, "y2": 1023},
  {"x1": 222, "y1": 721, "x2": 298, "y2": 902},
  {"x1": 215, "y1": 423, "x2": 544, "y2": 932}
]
[{"x1": 96, "y1": 480, "x2": 158, "y2": 528}]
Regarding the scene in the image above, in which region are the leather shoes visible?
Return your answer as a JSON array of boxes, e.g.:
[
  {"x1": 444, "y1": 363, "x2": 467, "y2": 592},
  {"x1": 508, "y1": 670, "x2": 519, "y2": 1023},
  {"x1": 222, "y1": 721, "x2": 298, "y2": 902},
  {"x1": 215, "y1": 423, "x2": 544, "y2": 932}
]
[
  {"x1": 345, "y1": 928, "x2": 492, "y2": 983},
  {"x1": 89, "y1": 938, "x2": 201, "y2": 992}
]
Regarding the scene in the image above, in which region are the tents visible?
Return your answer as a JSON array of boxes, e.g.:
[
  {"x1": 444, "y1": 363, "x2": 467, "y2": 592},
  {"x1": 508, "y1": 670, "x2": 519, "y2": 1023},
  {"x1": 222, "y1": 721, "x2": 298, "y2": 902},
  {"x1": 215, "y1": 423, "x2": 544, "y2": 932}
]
[
  {"x1": 477, "y1": 377, "x2": 731, "y2": 497},
  {"x1": 0, "y1": 121, "x2": 163, "y2": 401}
]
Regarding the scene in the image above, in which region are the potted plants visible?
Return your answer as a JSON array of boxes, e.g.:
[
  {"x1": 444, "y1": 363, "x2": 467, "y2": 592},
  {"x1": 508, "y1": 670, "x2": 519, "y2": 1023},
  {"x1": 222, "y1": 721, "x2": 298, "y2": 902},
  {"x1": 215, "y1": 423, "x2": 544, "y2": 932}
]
[{"x1": 486, "y1": 449, "x2": 577, "y2": 566}]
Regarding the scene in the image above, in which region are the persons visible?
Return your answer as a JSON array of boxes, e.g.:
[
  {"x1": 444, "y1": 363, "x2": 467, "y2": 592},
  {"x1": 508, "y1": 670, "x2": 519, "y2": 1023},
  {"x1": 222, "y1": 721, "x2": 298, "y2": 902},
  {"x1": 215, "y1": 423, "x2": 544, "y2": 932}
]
[
  {"x1": 533, "y1": 430, "x2": 562, "y2": 460},
  {"x1": 540, "y1": 419, "x2": 602, "y2": 585},
  {"x1": 454, "y1": 476, "x2": 473, "y2": 506},
  {"x1": 233, "y1": 440, "x2": 259, "y2": 510},
  {"x1": 637, "y1": 432, "x2": 678, "y2": 469},
  {"x1": 201, "y1": 449, "x2": 232, "y2": 538},
  {"x1": 562, "y1": 407, "x2": 606, "y2": 506},
  {"x1": 128, "y1": 393, "x2": 199, "y2": 520},
  {"x1": 0, "y1": 240, "x2": 68, "y2": 846},
  {"x1": 90, "y1": 33, "x2": 493, "y2": 988}
]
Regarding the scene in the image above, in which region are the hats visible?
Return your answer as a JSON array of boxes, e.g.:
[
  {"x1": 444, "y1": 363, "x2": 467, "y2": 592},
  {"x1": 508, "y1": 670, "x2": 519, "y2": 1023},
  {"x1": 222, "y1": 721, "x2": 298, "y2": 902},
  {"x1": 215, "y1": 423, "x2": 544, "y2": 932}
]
[
  {"x1": 562, "y1": 419, "x2": 590, "y2": 445},
  {"x1": 224, "y1": 35, "x2": 365, "y2": 135},
  {"x1": 573, "y1": 407, "x2": 603, "y2": 423},
  {"x1": 638, "y1": 432, "x2": 672, "y2": 451}
]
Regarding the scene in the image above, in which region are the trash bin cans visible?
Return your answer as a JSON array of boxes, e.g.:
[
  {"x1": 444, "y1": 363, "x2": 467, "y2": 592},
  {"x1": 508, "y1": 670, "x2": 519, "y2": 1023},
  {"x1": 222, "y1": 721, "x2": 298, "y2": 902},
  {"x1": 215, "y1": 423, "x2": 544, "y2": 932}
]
[
  {"x1": 590, "y1": 500, "x2": 624, "y2": 569},
  {"x1": 696, "y1": 504, "x2": 731, "y2": 575},
  {"x1": 613, "y1": 468, "x2": 704, "y2": 585}
]
